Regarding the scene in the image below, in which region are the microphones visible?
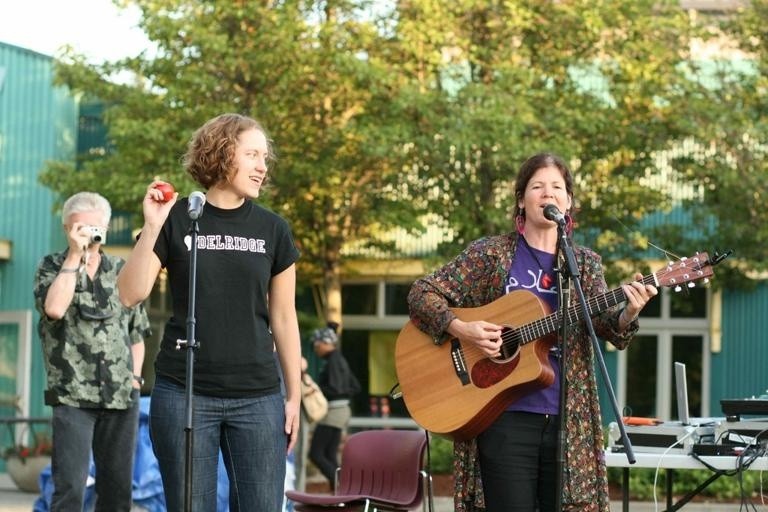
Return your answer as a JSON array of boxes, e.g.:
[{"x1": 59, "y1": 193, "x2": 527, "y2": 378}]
[
  {"x1": 188, "y1": 191, "x2": 206, "y2": 220},
  {"x1": 543, "y1": 204, "x2": 566, "y2": 226}
]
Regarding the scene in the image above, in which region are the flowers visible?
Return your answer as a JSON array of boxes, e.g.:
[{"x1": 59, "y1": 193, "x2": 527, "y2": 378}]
[{"x1": 3, "y1": 427, "x2": 52, "y2": 456}]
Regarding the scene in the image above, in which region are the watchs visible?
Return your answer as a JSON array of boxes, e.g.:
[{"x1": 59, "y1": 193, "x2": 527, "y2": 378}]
[{"x1": 134, "y1": 375, "x2": 145, "y2": 386}]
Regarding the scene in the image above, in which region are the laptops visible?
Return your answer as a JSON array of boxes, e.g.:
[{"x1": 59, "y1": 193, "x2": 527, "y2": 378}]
[{"x1": 674, "y1": 362, "x2": 744, "y2": 426}]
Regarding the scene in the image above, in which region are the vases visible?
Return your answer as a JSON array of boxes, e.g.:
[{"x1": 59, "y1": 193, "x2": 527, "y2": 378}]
[{"x1": 5, "y1": 458, "x2": 51, "y2": 493}]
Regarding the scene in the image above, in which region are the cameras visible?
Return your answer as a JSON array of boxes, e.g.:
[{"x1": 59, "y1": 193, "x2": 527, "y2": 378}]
[{"x1": 80, "y1": 226, "x2": 107, "y2": 245}]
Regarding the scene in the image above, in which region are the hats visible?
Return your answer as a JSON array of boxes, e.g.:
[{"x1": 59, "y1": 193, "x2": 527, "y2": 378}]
[{"x1": 309, "y1": 327, "x2": 338, "y2": 346}]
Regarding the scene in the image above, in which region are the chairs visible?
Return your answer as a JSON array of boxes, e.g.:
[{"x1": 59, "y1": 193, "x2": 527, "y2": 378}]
[{"x1": 284, "y1": 430, "x2": 436, "y2": 512}]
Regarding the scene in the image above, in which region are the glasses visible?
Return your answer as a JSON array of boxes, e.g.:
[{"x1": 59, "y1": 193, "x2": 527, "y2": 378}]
[{"x1": 75, "y1": 284, "x2": 114, "y2": 322}]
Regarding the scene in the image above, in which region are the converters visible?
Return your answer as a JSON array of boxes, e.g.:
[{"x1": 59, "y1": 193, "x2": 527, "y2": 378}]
[{"x1": 694, "y1": 444, "x2": 734, "y2": 456}]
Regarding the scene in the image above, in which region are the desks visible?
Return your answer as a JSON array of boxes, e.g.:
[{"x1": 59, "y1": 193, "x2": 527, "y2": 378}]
[{"x1": 604, "y1": 441, "x2": 768, "y2": 512}]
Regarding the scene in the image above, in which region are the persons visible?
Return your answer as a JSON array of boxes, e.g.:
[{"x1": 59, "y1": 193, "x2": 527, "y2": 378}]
[
  {"x1": 34, "y1": 192, "x2": 153, "y2": 512},
  {"x1": 117, "y1": 113, "x2": 302, "y2": 512},
  {"x1": 270, "y1": 342, "x2": 308, "y2": 404},
  {"x1": 406, "y1": 152, "x2": 660, "y2": 512},
  {"x1": 308, "y1": 326, "x2": 362, "y2": 492}
]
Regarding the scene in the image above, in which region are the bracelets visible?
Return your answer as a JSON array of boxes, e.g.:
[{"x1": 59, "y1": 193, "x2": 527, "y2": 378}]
[
  {"x1": 59, "y1": 268, "x2": 80, "y2": 273},
  {"x1": 621, "y1": 310, "x2": 639, "y2": 325}
]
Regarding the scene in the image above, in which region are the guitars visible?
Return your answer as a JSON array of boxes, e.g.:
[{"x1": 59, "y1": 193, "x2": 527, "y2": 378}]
[
  {"x1": 395, "y1": 248, "x2": 733, "y2": 441},
  {"x1": 301, "y1": 371, "x2": 329, "y2": 424}
]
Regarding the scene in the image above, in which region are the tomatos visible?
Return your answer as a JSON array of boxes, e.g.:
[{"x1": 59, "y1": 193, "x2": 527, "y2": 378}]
[{"x1": 153, "y1": 184, "x2": 175, "y2": 202}]
